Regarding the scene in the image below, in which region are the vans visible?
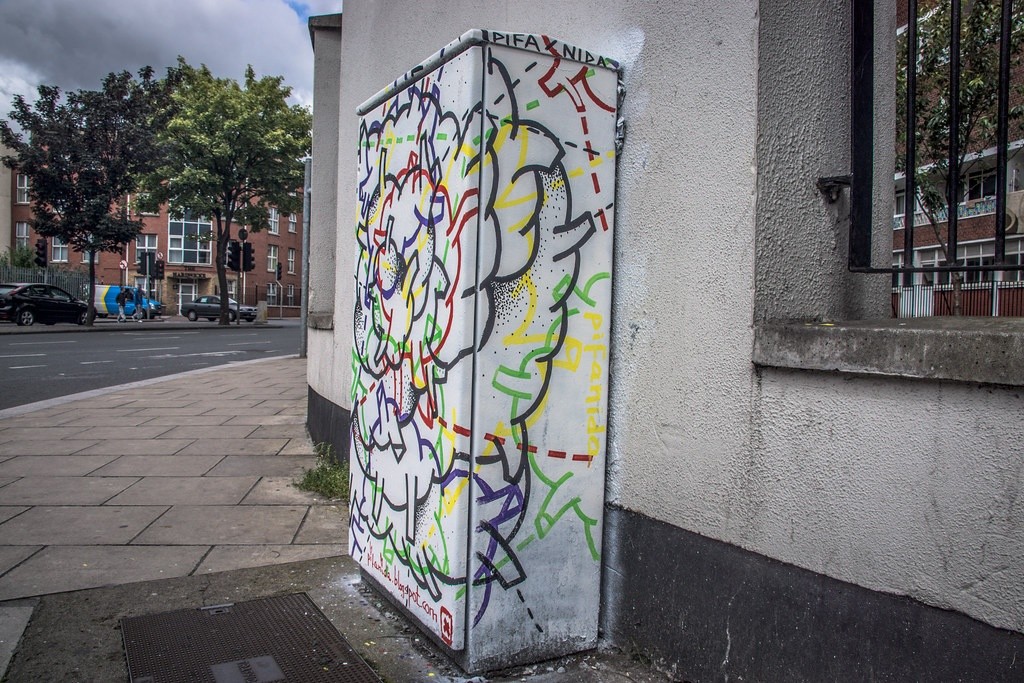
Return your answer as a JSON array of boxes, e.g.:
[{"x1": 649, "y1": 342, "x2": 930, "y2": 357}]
[{"x1": 91, "y1": 285, "x2": 163, "y2": 319}]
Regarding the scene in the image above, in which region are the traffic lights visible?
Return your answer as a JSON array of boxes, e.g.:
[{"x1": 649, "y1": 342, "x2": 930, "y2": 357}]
[
  {"x1": 34, "y1": 238, "x2": 48, "y2": 266},
  {"x1": 277, "y1": 262, "x2": 282, "y2": 281},
  {"x1": 226, "y1": 242, "x2": 241, "y2": 270},
  {"x1": 243, "y1": 242, "x2": 256, "y2": 272},
  {"x1": 137, "y1": 251, "x2": 146, "y2": 275},
  {"x1": 156, "y1": 260, "x2": 165, "y2": 279}
]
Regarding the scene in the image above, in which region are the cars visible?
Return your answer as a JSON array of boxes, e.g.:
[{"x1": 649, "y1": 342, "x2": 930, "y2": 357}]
[
  {"x1": 0, "y1": 281, "x2": 98, "y2": 326},
  {"x1": 180, "y1": 294, "x2": 258, "y2": 323}
]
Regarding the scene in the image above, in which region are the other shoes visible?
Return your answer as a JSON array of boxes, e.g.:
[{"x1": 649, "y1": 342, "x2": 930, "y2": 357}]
[
  {"x1": 132, "y1": 316, "x2": 136, "y2": 321},
  {"x1": 118, "y1": 319, "x2": 120, "y2": 323},
  {"x1": 123, "y1": 320, "x2": 126, "y2": 323},
  {"x1": 138, "y1": 320, "x2": 142, "y2": 323}
]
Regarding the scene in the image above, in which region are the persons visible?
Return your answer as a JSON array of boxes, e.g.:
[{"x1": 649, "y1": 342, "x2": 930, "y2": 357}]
[
  {"x1": 115, "y1": 284, "x2": 128, "y2": 324},
  {"x1": 131, "y1": 284, "x2": 147, "y2": 323}
]
